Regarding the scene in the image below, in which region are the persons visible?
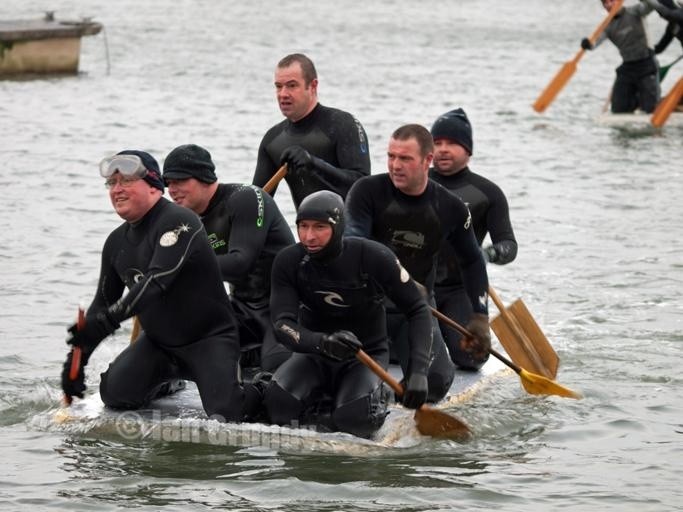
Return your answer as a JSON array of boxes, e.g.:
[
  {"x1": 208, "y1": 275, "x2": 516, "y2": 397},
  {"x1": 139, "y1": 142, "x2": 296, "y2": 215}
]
[
  {"x1": 160, "y1": 143, "x2": 296, "y2": 356},
  {"x1": 579, "y1": 0, "x2": 683, "y2": 114},
  {"x1": 640, "y1": 0, "x2": 683, "y2": 55},
  {"x1": 341, "y1": 124, "x2": 492, "y2": 404},
  {"x1": 263, "y1": 190, "x2": 434, "y2": 438},
  {"x1": 60, "y1": 148, "x2": 275, "y2": 424},
  {"x1": 428, "y1": 107, "x2": 519, "y2": 372},
  {"x1": 249, "y1": 51, "x2": 372, "y2": 212}
]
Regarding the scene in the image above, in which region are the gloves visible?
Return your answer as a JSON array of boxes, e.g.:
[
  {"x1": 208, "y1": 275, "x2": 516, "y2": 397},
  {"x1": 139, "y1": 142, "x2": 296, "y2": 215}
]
[
  {"x1": 579, "y1": 35, "x2": 594, "y2": 53},
  {"x1": 276, "y1": 146, "x2": 322, "y2": 181},
  {"x1": 322, "y1": 327, "x2": 362, "y2": 363},
  {"x1": 396, "y1": 353, "x2": 432, "y2": 405},
  {"x1": 461, "y1": 317, "x2": 493, "y2": 367},
  {"x1": 66, "y1": 308, "x2": 120, "y2": 364},
  {"x1": 58, "y1": 352, "x2": 87, "y2": 401}
]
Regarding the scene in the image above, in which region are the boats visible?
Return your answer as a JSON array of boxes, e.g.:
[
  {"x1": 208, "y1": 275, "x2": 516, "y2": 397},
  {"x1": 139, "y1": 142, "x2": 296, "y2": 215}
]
[
  {"x1": 27, "y1": 308, "x2": 547, "y2": 457},
  {"x1": 589, "y1": 93, "x2": 682, "y2": 134}
]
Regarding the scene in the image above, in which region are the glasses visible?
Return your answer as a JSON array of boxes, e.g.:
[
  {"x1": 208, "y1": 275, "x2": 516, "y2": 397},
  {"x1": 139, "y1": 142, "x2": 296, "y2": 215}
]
[{"x1": 96, "y1": 155, "x2": 146, "y2": 181}]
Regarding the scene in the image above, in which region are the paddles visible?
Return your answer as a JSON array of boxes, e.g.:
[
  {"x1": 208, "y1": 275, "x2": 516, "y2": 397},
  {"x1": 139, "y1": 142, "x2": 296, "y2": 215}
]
[
  {"x1": 651, "y1": 73, "x2": 683, "y2": 130},
  {"x1": 659, "y1": 54, "x2": 683, "y2": 79},
  {"x1": 426, "y1": 305, "x2": 583, "y2": 399},
  {"x1": 355, "y1": 348, "x2": 476, "y2": 444},
  {"x1": 48, "y1": 300, "x2": 87, "y2": 428},
  {"x1": 530, "y1": 0, "x2": 627, "y2": 115},
  {"x1": 488, "y1": 283, "x2": 560, "y2": 390}
]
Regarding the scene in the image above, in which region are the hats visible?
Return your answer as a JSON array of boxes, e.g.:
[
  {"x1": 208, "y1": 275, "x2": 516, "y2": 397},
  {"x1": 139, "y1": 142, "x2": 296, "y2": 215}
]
[
  {"x1": 107, "y1": 151, "x2": 164, "y2": 191},
  {"x1": 296, "y1": 191, "x2": 346, "y2": 261},
  {"x1": 429, "y1": 107, "x2": 474, "y2": 153},
  {"x1": 164, "y1": 143, "x2": 217, "y2": 182}
]
